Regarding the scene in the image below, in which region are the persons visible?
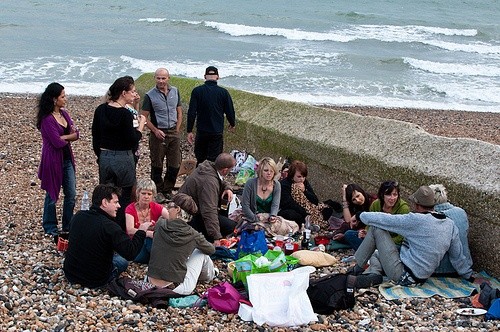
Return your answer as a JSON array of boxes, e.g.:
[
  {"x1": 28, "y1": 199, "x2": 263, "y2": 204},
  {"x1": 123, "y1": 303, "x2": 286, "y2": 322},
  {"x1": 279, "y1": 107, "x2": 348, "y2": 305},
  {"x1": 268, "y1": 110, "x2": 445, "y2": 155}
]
[
  {"x1": 179, "y1": 153, "x2": 239, "y2": 244},
  {"x1": 142, "y1": 68, "x2": 182, "y2": 204},
  {"x1": 63, "y1": 182, "x2": 153, "y2": 290},
  {"x1": 124, "y1": 178, "x2": 219, "y2": 296},
  {"x1": 342, "y1": 201, "x2": 349, "y2": 208},
  {"x1": 35, "y1": 82, "x2": 80, "y2": 237},
  {"x1": 333, "y1": 183, "x2": 377, "y2": 241},
  {"x1": 242, "y1": 157, "x2": 281, "y2": 224},
  {"x1": 358, "y1": 180, "x2": 410, "y2": 251},
  {"x1": 348, "y1": 183, "x2": 476, "y2": 288},
  {"x1": 187, "y1": 66, "x2": 236, "y2": 169},
  {"x1": 91, "y1": 75, "x2": 147, "y2": 231},
  {"x1": 280, "y1": 160, "x2": 344, "y2": 229}
]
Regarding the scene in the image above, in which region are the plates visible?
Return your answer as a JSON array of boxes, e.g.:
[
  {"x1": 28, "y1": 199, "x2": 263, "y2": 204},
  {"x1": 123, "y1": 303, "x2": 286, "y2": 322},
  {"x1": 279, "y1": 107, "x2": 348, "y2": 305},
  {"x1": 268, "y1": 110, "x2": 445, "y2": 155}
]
[{"x1": 456, "y1": 308, "x2": 488, "y2": 315}]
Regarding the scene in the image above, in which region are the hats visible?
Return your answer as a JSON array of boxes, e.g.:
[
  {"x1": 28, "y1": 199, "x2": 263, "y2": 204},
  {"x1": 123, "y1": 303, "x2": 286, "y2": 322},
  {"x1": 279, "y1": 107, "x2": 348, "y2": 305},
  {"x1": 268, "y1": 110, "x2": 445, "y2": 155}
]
[
  {"x1": 206, "y1": 66, "x2": 218, "y2": 76},
  {"x1": 165, "y1": 193, "x2": 198, "y2": 215},
  {"x1": 408, "y1": 186, "x2": 437, "y2": 206}
]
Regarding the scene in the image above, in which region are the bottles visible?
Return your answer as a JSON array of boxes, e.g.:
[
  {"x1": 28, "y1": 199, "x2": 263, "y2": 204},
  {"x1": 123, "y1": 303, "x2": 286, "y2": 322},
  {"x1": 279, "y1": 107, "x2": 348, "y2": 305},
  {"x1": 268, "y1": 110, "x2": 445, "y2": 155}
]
[
  {"x1": 316, "y1": 243, "x2": 326, "y2": 252},
  {"x1": 272, "y1": 241, "x2": 294, "y2": 256},
  {"x1": 301, "y1": 231, "x2": 309, "y2": 250},
  {"x1": 288, "y1": 228, "x2": 293, "y2": 237},
  {"x1": 81, "y1": 191, "x2": 90, "y2": 210},
  {"x1": 342, "y1": 255, "x2": 356, "y2": 263}
]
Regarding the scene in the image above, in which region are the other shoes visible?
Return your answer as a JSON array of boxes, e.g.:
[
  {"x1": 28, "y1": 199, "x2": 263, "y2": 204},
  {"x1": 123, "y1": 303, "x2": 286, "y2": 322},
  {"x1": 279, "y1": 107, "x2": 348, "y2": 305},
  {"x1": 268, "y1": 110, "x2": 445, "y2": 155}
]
[
  {"x1": 50, "y1": 231, "x2": 60, "y2": 238},
  {"x1": 345, "y1": 266, "x2": 383, "y2": 288}
]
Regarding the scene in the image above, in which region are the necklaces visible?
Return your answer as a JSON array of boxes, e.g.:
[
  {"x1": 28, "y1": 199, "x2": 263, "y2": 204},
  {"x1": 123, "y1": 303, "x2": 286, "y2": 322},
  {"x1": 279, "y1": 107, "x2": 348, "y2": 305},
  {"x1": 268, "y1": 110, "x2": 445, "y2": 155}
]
[
  {"x1": 116, "y1": 100, "x2": 124, "y2": 107},
  {"x1": 260, "y1": 180, "x2": 273, "y2": 193},
  {"x1": 53, "y1": 111, "x2": 60, "y2": 115},
  {"x1": 137, "y1": 203, "x2": 149, "y2": 222}
]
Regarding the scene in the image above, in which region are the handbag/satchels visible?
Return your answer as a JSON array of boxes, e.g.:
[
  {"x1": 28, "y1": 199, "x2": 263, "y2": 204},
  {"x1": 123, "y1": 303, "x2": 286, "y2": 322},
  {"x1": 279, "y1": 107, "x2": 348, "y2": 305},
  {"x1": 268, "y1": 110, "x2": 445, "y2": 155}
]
[
  {"x1": 245, "y1": 265, "x2": 320, "y2": 327},
  {"x1": 227, "y1": 249, "x2": 299, "y2": 295},
  {"x1": 193, "y1": 281, "x2": 247, "y2": 314},
  {"x1": 306, "y1": 272, "x2": 378, "y2": 316},
  {"x1": 235, "y1": 223, "x2": 269, "y2": 256},
  {"x1": 230, "y1": 149, "x2": 289, "y2": 186},
  {"x1": 288, "y1": 249, "x2": 337, "y2": 267}
]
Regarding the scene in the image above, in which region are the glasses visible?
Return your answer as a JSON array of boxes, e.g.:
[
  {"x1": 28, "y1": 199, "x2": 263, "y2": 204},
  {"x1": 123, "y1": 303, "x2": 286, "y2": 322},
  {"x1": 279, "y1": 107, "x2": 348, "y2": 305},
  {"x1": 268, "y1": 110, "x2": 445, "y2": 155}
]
[
  {"x1": 384, "y1": 180, "x2": 399, "y2": 186},
  {"x1": 165, "y1": 205, "x2": 176, "y2": 210}
]
